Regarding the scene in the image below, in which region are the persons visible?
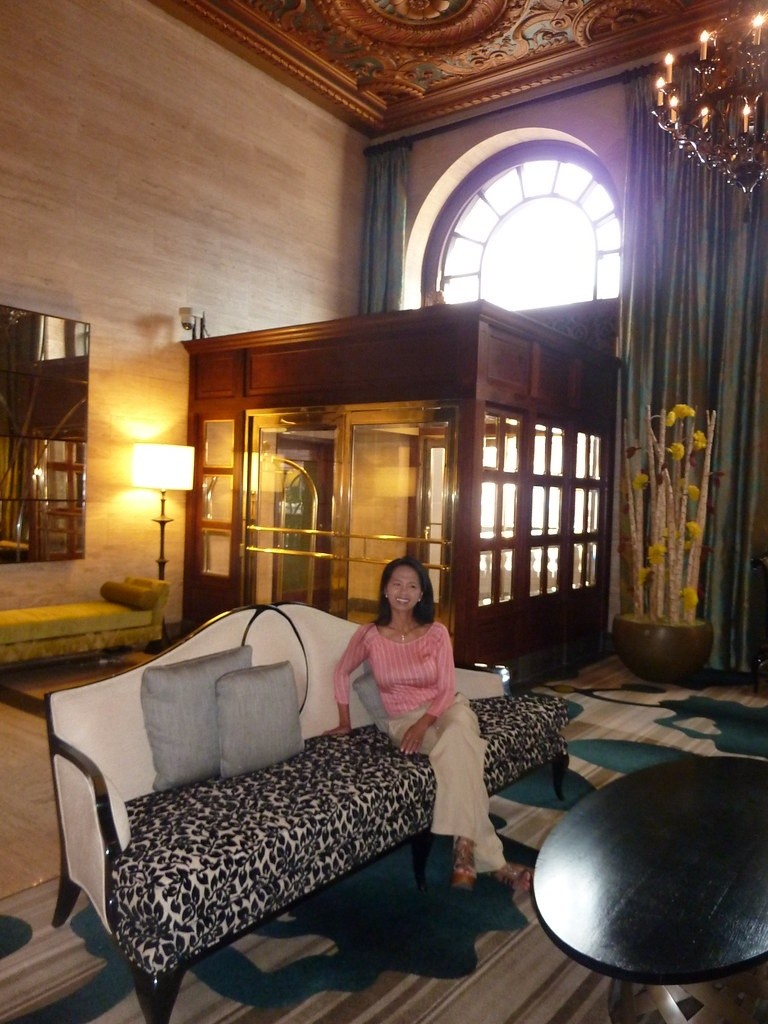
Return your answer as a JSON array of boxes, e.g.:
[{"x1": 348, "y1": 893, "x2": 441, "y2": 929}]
[{"x1": 324, "y1": 557, "x2": 533, "y2": 893}]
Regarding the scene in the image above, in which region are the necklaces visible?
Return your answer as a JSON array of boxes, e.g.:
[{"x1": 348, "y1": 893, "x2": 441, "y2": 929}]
[{"x1": 400, "y1": 634, "x2": 405, "y2": 640}]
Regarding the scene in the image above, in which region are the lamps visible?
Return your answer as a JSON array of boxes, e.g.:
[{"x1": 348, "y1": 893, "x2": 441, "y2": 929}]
[
  {"x1": 651, "y1": 0, "x2": 768, "y2": 224},
  {"x1": 132, "y1": 443, "x2": 195, "y2": 653}
]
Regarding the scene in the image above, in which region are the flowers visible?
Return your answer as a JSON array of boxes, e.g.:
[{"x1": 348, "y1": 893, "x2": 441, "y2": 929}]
[{"x1": 615, "y1": 403, "x2": 719, "y2": 625}]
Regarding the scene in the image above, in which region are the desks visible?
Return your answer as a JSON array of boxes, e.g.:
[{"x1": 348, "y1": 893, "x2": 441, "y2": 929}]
[{"x1": 530, "y1": 757, "x2": 768, "y2": 1024}]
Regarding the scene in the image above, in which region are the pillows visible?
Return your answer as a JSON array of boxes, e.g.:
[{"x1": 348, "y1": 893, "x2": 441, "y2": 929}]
[
  {"x1": 140, "y1": 645, "x2": 252, "y2": 793},
  {"x1": 214, "y1": 661, "x2": 304, "y2": 779},
  {"x1": 100, "y1": 581, "x2": 156, "y2": 610}
]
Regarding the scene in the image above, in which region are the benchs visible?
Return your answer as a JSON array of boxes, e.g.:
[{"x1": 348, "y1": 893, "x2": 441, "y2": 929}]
[
  {"x1": 0, "y1": 577, "x2": 171, "y2": 665},
  {"x1": 43, "y1": 599, "x2": 569, "y2": 1024}
]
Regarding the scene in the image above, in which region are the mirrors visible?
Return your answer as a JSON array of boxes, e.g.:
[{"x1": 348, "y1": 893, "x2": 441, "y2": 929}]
[{"x1": 0, "y1": 303, "x2": 90, "y2": 564}]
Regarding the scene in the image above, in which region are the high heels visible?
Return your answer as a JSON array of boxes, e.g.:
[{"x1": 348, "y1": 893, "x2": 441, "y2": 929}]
[
  {"x1": 479, "y1": 864, "x2": 534, "y2": 891},
  {"x1": 452, "y1": 835, "x2": 477, "y2": 890}
]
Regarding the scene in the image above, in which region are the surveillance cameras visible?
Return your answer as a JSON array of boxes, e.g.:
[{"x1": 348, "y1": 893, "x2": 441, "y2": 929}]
[{"x1": 181, "y1": 315, "x2": 194, "y2": 330}]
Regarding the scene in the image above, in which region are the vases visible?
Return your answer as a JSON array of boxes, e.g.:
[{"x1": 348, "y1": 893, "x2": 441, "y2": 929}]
[{"x1": 612, "y1": 611, "x2": 716, "y2": 685}]
